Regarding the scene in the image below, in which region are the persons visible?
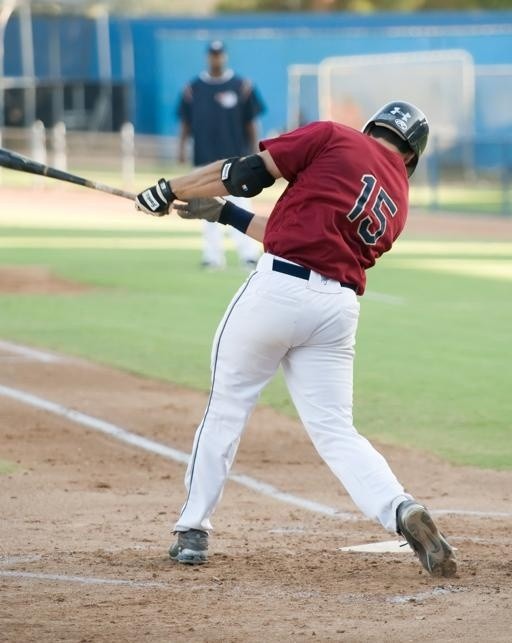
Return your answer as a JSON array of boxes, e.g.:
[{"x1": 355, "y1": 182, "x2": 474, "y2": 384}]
[
  {"x1": 176, "y1": 38, "x2": 264, "y2": 269},
  {"x1": 134, "y1": 100, "x2": 458, "y2": 579}
]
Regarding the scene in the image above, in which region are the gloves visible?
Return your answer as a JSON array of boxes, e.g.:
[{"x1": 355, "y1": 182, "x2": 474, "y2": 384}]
[
  {"x1": 133, "y1": 177, "x2": 176, "y2": 217},
  {"x1": 178, "y1": 190, "x2": 229, "y2": 224}
]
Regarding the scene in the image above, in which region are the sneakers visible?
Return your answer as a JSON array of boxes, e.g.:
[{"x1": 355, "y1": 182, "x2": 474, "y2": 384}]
[
  {"x1": 392, "y1": 496, "x2": 462, "y2": 580},
  {"x1": 165, "y1": 531, "x2": 208, "y2": 566}
]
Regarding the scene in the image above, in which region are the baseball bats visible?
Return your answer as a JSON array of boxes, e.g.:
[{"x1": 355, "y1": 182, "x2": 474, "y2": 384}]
[{"x1": 0, "y1": 147, "x2": 138, "y2": 202}]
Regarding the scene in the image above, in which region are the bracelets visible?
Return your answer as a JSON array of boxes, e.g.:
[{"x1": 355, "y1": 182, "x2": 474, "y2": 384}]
[{"x1": 218, "y1": 201, "x2": 255, "y2": 234}]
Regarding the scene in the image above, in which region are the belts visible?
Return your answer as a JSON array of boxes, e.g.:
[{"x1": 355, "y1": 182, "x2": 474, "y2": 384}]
[{"x1": 270, "y1": 258, "x2": 358, "y2": 296}]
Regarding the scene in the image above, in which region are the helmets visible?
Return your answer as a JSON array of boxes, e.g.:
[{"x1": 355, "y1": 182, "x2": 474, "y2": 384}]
[{"x1": 361, "y1": 99, "x2": 431, "y2": 177}]
